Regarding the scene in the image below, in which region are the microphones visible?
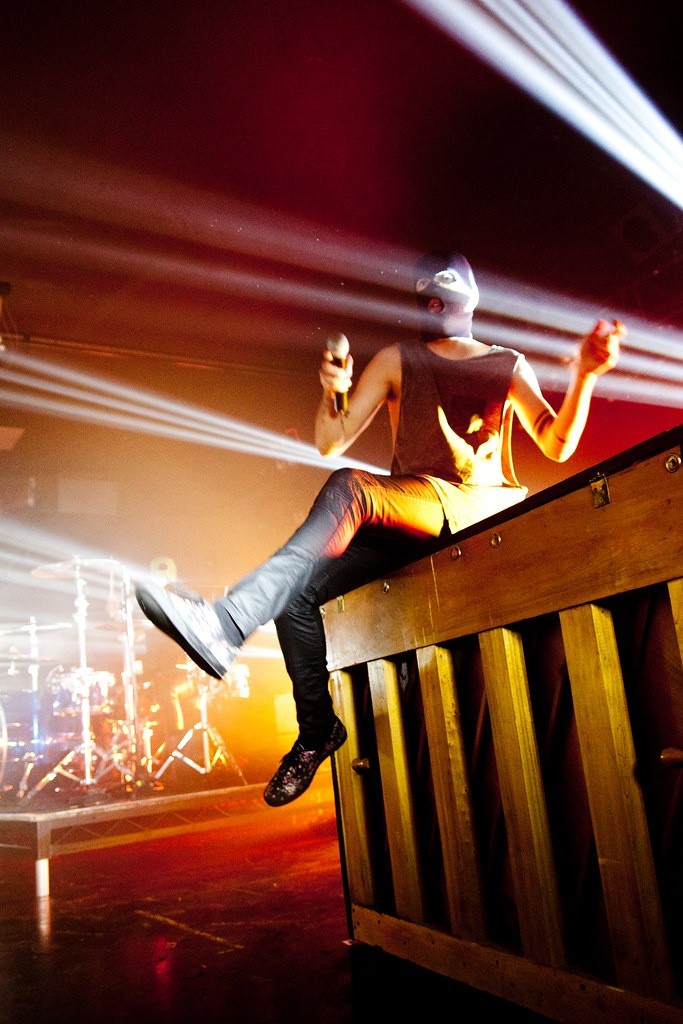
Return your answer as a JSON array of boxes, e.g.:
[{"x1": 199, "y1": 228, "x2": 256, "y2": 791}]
[{"x1": 326, "y1": 332, "x2": 348, "y2": 415}]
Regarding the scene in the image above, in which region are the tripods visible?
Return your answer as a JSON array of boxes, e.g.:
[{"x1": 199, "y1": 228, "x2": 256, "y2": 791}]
[{"x1": 14, "y1": 573, "x2": 249, "y2": 807}]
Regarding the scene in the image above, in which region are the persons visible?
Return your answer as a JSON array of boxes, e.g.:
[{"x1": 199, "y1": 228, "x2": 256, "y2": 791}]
[
  {"x1": 133, "y1": 243, "x2": 627, "y2": 810},
  {"x1": 142, "y1": 555, "x2": 203, "y2": 736}
]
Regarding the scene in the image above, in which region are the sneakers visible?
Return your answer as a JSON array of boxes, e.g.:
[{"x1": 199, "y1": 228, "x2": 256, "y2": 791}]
[
  {"x1": 263, "y1": 716, "x2": 348, "y2": 807},
  {"x1": 136, "y1": 579, "x2": 243, "y2": 679}
]
